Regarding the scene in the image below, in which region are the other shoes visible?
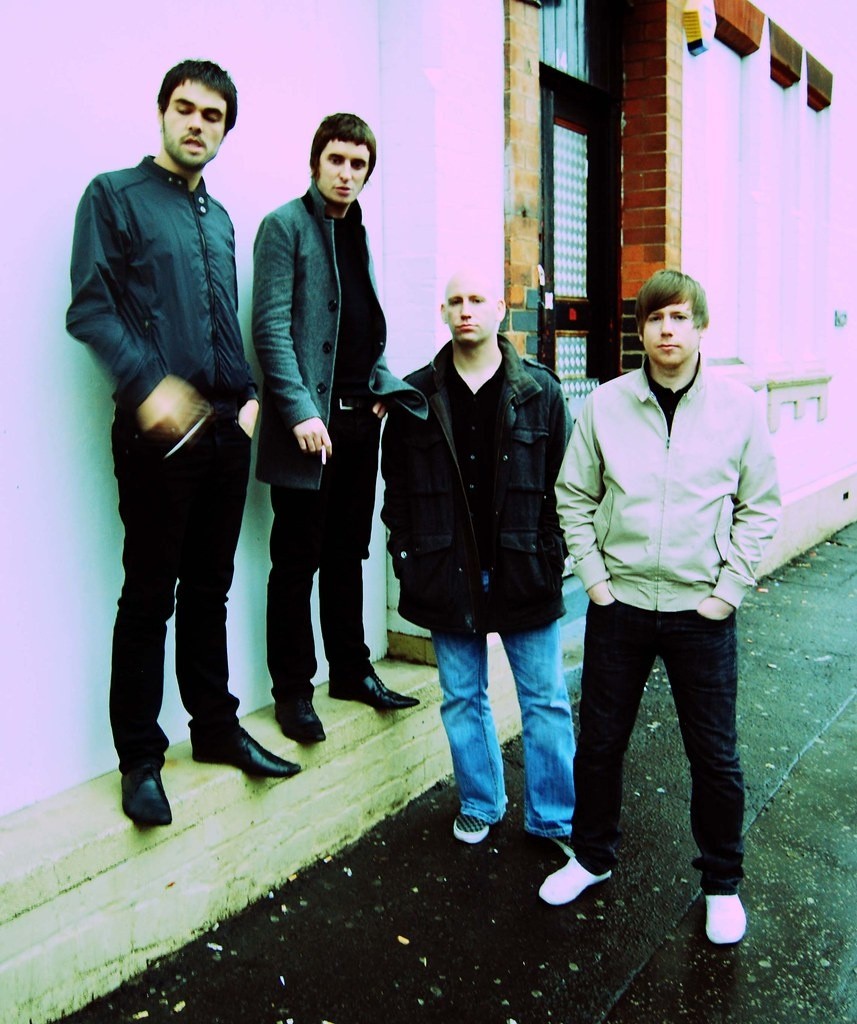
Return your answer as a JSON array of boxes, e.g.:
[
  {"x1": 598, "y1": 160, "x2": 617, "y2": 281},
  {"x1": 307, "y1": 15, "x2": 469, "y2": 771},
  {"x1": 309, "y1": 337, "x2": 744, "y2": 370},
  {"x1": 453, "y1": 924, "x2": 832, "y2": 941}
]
[
  {"x1": 551, "y1": 835, "x2": 575, "y2": 859},
  {"x1": 453, "y1": 814, "x2": 489, "y2": 844},
  {"x1": 539, "y1": 857, "x2": 612, "y2": 905},
  {"x1": 705, "y1": 893, "x2": 746, "y2": 943}
]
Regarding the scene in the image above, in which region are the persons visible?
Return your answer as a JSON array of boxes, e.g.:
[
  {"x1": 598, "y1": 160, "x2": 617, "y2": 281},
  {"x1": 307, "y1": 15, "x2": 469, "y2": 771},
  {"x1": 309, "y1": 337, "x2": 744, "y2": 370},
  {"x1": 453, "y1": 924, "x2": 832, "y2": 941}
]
[
  {"x1": 65, "y1": 58, "x2": 303, "y2": 828},
  {"x1": 381, "y1": 264, "x2": 576, "y2": 857},
  {"x1": 249, "y1": 113, "x2": 428, "y2": 744},
  {"x1": 538, "y1": 267, "x2": 784, "y2": 947}
]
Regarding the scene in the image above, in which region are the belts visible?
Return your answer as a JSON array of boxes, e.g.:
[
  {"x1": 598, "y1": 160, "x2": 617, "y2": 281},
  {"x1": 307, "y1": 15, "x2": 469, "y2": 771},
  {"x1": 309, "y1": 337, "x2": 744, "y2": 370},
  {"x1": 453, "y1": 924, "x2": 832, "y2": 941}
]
[{"x1": 330, "y1": 399, "x2": 371, "y2": 411}]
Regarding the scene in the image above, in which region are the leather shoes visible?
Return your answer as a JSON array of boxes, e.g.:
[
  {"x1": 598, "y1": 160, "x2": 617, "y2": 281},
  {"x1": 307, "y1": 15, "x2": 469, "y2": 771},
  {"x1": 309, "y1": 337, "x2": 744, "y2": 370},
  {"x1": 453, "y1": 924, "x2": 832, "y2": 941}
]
[
  {"x1": 275, "y1": 697, "x2": 326, "y2": 745},
  {"x1": 121, "y1": 767, "x2": 172, "y2": 828},
  {"x1": 328, "y1": 668, "x2": 420, "y2": 710},
  {"x1": 192, "y1": 726, "x2": 301, "y2": 777}
]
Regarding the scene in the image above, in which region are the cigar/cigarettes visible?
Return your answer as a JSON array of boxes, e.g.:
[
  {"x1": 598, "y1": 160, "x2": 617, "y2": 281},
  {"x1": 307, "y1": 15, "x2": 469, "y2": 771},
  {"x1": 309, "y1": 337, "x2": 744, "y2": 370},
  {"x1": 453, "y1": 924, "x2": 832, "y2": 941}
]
[{"x1": 321, "y1": 445, "x2": 327, "y2": 465}]
